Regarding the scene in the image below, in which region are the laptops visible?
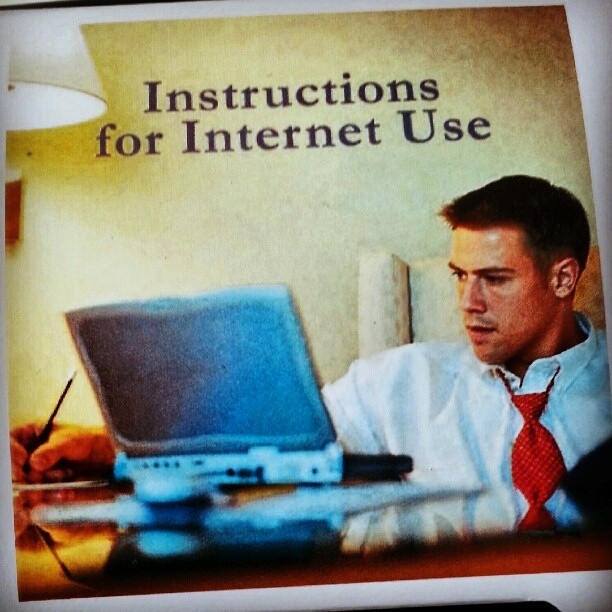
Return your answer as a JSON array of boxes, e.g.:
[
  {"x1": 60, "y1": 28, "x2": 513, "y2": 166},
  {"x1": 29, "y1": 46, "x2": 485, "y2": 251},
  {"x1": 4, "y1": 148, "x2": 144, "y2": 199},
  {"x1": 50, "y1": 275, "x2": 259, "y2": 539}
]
[{"x1": 64, "y1": 283, "x2": 413, "y2": 483}]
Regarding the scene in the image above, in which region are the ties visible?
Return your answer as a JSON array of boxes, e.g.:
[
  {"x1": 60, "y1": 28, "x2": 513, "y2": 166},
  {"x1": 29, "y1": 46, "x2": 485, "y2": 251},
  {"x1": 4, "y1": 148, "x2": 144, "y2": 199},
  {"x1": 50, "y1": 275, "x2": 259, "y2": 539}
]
[{"x1": 497, "y1": 366, "x2": 567, "y2": 534}]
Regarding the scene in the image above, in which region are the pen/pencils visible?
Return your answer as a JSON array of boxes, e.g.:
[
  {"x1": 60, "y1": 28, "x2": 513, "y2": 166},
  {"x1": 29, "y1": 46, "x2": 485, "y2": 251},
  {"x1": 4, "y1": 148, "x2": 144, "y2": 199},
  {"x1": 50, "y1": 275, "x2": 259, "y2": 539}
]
[{"x1": 20, "y1": 371, "x2": 77, "y2": 475}]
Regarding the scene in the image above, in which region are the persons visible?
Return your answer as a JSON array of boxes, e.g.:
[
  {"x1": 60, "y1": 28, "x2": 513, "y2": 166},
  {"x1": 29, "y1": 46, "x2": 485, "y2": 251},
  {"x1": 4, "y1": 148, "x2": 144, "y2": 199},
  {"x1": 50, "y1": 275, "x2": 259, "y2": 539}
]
[
  {"x1": 12, "y1": 486, "x2": 588, "y2": 556},
  {"x1": 11, "y1": 175, "x2": 612, "y2": 484}
]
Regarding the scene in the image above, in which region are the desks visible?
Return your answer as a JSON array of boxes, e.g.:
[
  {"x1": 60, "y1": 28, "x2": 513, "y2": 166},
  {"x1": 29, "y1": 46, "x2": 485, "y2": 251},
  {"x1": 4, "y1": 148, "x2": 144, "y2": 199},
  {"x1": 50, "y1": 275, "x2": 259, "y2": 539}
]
[{"x1": 10, "y1": 452, "x2": 607, "y2": 602}]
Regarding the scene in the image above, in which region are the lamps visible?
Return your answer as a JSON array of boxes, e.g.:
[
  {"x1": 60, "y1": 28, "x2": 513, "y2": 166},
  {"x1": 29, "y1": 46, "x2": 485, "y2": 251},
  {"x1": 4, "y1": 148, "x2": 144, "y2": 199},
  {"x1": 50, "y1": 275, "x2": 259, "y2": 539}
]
[{"x1": 3, "y1": 21, "x2": 108, "y2": 241}]
